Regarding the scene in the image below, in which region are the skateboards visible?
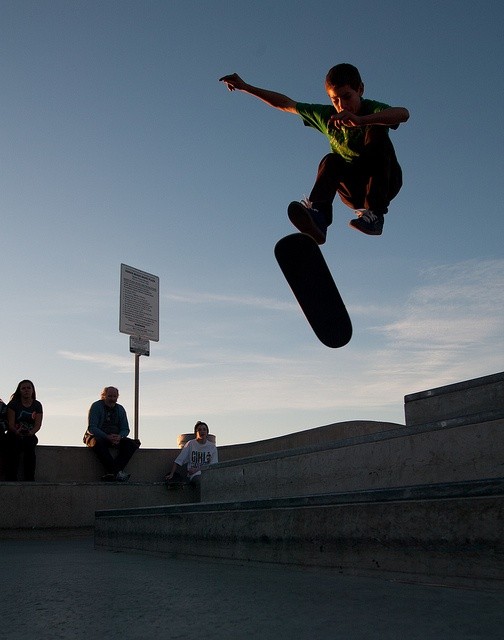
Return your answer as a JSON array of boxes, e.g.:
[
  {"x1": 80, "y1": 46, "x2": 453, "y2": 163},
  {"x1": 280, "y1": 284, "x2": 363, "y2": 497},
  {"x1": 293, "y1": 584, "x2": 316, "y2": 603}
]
[{"x1": 274, "y1": 232, "x2": 354, "y2": 349}]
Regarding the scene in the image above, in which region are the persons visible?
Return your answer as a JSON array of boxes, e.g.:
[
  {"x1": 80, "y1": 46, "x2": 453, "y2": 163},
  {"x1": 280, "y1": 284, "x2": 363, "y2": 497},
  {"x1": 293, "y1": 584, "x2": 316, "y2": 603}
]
[
  {"x1": 218, "y1": 63, "x2": 410, "y2": 245},
  {"x1": 165, "y1": 422, "x2": 219, "y2": 502},
  {"x1": 7, "y1": 380, "x2": 43, "y2": 481},
  {"x1": 88, "y1": 386, "x2": 137, "y2": 482}
]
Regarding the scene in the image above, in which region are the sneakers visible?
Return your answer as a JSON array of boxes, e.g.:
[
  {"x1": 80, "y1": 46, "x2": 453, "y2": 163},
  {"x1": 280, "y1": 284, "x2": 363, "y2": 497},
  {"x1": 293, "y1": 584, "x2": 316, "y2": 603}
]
[
  {"x1": 349, "y1": 209, "x2": 384, "y2": 235},
  {"x1": 287, "y1": 197, "x2": 327, "y2": 244},
  {"x1": 117, "y1": 470, "x2": 131, "y2": 480}
]
